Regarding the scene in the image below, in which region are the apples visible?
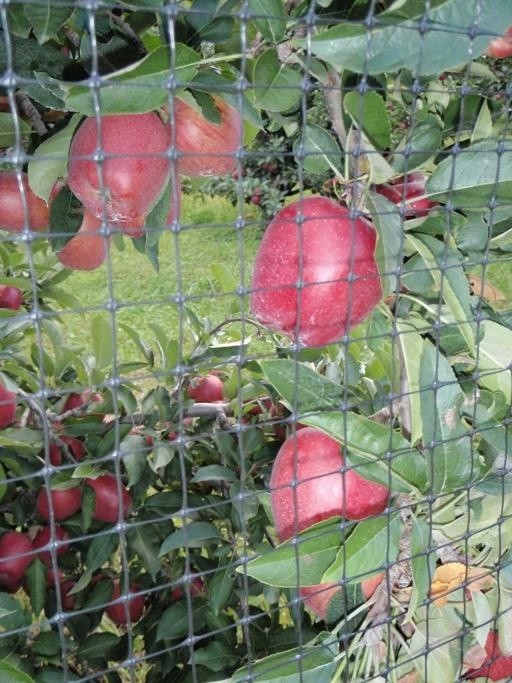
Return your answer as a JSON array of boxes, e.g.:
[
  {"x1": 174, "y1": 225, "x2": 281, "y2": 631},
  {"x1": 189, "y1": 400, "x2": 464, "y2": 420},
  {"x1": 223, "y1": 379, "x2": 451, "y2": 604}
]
[
  {"x1": 158, "y1": 97, "x2": 240, "y2": 176},
  {"x1": 113, "y1": 174, "x2": 181, "y2": 236},
  {"x1": 461, "y1": 629, "x2": 512, "y2": 681},
  {"x1": 485, "y1": 26, "x2": 512, "y2": 58},
  {"x1": 0, "y1": 369, "x2": 389, "y2": 622},
  {"x1": 67, "y1": 111, "x2": 172, "y2": 221},
  {"x1": 0, "y1": 170, "x2": 58, "y2": 232},
  {"x1": 0, "y1": 284, "x2": 21, "y2": 309},
  {"x1": 48, "y1": 181, "x2": 111, "y2": 270},
  {"x1": 233, "y1": 162, "x2": 441, "y2": 347}
]
